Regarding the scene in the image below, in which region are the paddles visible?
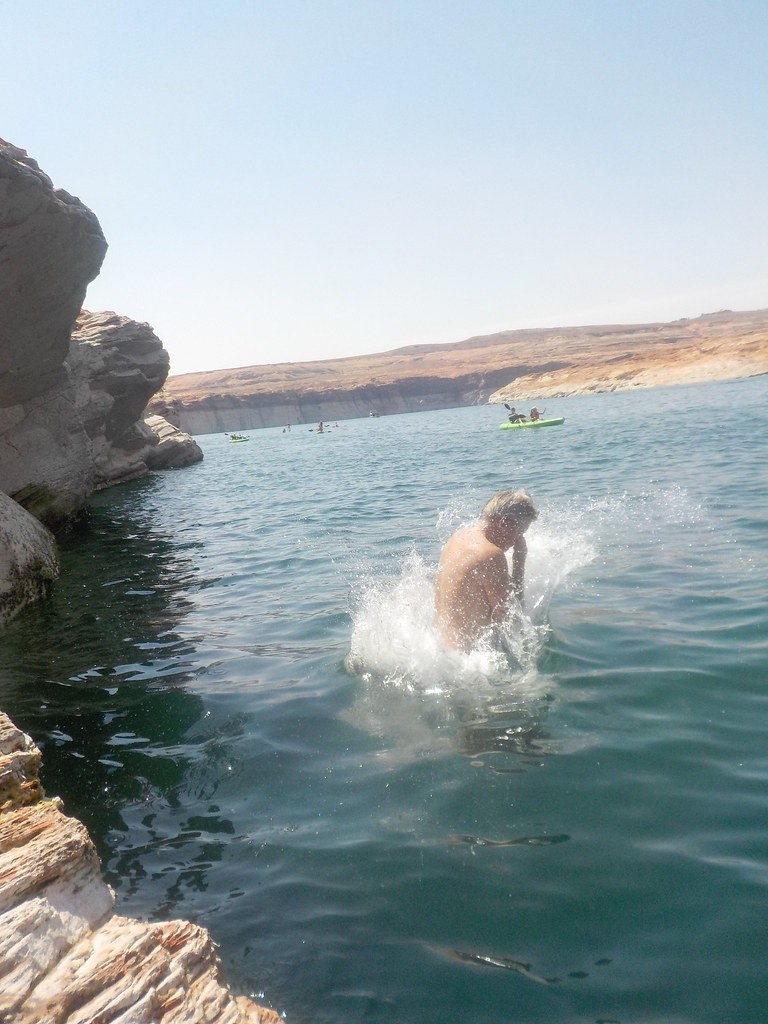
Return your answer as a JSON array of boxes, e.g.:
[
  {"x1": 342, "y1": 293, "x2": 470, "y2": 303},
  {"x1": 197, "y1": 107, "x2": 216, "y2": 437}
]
[{"x1": 504, "y1": 403, "x2": 511, "y2": 410}]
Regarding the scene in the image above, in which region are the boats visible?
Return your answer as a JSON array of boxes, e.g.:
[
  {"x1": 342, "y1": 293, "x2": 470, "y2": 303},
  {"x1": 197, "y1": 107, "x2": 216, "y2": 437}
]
[
  {"x1": 229, "y1": 436, "x2": 249, "y2": 443},
  {"x1": 499, "y1": 417, "x2": 566, "y2": 430}
]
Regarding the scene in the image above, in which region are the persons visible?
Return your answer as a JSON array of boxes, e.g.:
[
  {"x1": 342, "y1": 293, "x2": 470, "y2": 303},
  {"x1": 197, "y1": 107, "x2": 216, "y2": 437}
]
[
  {"x1": 230, "y1": 422, "x2": 337, "y2": 440},
  {"x1": 530, "y1": 407, "x2": 546, "y2": 422},
  {"x1": 434, "y1": 489, "x2": 540, "y2": 655},
  {"x1": 509, "y1": 408, "x2": 527, "y2": 423}
]
